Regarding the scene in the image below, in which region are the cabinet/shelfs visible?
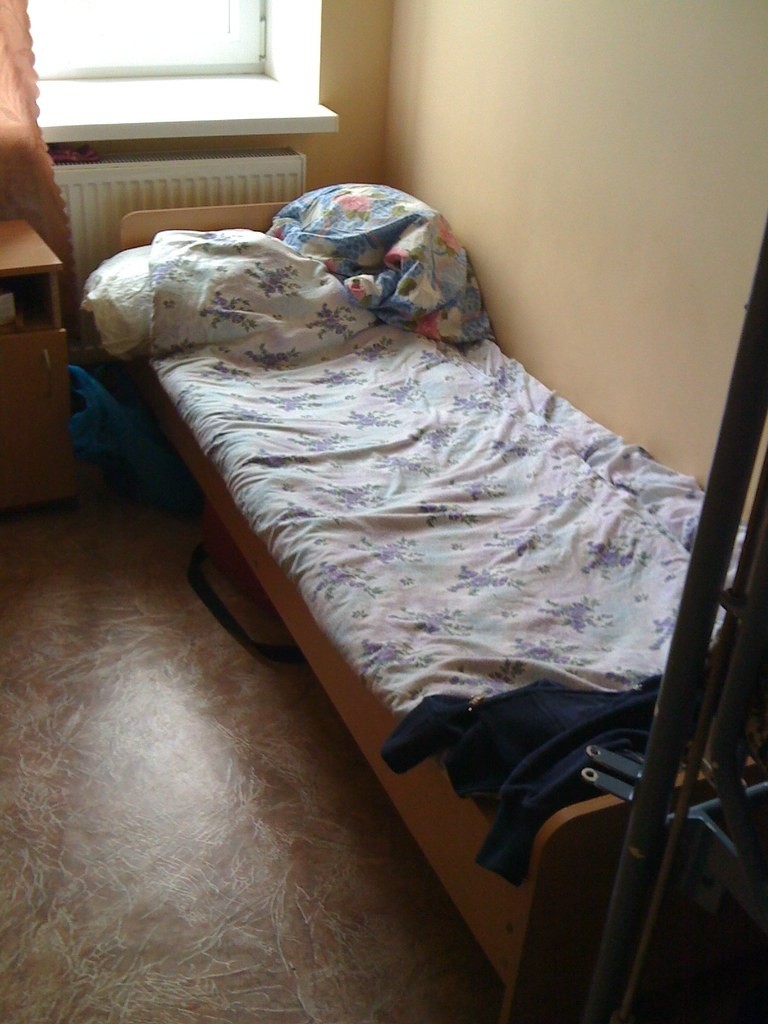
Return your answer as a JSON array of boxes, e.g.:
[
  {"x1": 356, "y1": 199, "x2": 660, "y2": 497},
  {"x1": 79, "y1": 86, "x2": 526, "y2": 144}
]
[{"x1": 0, "y1": 217, "x2": 96, "y2": 522}]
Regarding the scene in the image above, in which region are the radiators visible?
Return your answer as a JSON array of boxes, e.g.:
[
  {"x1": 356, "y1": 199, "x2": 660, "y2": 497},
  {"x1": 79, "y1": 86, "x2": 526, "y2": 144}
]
[{"x1": 48, "y1": 146, "x2": 311, "y2": 314}]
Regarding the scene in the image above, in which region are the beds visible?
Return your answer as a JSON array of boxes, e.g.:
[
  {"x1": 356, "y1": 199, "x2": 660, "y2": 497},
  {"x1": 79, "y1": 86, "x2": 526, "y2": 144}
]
[{"x1": 118, "y1": 189, "x2": 768, "y2": 1017}]
